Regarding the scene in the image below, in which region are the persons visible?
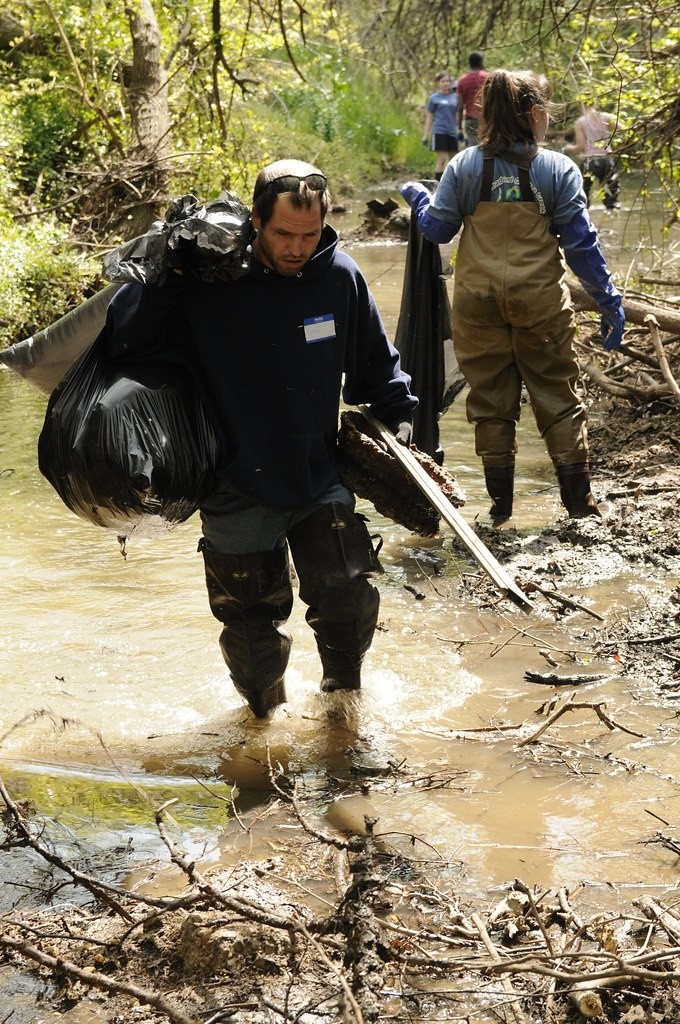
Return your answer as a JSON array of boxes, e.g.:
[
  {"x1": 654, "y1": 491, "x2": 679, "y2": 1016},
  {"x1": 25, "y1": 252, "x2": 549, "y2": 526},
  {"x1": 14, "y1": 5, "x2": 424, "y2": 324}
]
[
  {"x1": 422, "y1": 53, "x2": 492, "y2": 182},
  {"x1": 400, "y1": 67, "x2": 626, "y2": 519},
  {"x1": 105, "y1": 159, "x2": 420, "y2": 719},
  {"x1": 560, "y1": 101, "x2": 627, "y2": 211}
]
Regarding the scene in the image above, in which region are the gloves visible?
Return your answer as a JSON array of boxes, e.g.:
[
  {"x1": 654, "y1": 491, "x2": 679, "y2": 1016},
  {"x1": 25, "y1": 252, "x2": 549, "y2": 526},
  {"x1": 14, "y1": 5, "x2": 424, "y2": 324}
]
[
  {"x1": 456, "y1": 127, "x2": 466, "y2": 142},
  {"x1": 400, "y1": 181, "x2": 461, "y2": 244},
  {"x1": 548, "y1": 209, "x2": 626, "y2": 353},
  {"x1": 380, "y1": 417, "x2": 412, "y2": 446}
]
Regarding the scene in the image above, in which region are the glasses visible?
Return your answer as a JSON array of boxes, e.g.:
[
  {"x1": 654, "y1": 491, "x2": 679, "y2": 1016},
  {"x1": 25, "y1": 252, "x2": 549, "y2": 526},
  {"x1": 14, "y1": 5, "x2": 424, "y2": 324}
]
[{"x1": 258, "y1": 174, "x2": 330, "y2": 196}]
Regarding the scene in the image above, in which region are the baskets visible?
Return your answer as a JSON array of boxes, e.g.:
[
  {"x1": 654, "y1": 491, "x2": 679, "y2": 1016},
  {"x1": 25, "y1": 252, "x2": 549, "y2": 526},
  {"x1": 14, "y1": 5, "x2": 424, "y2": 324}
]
[{"x1": 335, "y1": 410, "x2": 467, "y2": 539}]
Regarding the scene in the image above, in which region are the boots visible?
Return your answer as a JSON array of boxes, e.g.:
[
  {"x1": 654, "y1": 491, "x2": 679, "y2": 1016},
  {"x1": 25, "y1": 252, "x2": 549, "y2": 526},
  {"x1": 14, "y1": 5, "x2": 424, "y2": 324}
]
[
  {"x1": 483, "y1": 466, "x2": 515, "y2": 521},
  {"x1": 555, "y1": 463, "x2": 603, "y2": 519}
]
